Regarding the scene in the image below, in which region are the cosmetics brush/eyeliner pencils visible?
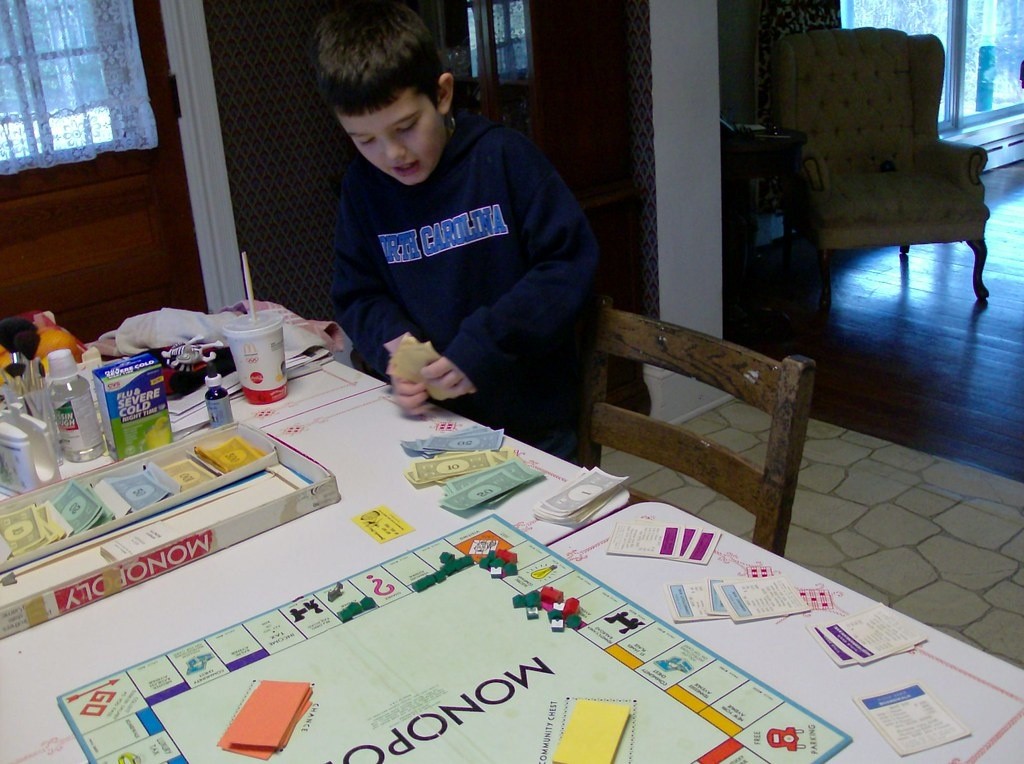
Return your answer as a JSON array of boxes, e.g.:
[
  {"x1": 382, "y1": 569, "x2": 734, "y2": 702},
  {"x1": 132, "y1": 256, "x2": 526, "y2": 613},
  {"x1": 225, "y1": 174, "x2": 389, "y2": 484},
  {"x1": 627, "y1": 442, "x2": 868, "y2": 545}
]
[{"x1": 0, "y1": 318, "x2": 54, "y2": 438}]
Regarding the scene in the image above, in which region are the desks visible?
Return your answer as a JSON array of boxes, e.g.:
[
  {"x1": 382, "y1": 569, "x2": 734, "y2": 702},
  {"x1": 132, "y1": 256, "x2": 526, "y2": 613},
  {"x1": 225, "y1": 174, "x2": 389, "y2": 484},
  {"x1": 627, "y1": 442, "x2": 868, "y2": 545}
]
[
  {"x1": 722, "y1": 128, "x2": 808, "y2": 328},
  {"x1": 0, "y1": 361, "x2": 1024, "y2": 764}
]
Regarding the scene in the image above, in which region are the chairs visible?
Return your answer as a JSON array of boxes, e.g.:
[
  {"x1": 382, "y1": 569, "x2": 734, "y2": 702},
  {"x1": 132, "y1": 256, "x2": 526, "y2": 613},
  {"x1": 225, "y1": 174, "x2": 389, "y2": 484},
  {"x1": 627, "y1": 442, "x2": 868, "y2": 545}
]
[
  {"x1": 773, "y1": 27, "x2": 990, "y2": 309},
  {"x1": 593, "y1": 295, "x2": 816, "y2": 555}
]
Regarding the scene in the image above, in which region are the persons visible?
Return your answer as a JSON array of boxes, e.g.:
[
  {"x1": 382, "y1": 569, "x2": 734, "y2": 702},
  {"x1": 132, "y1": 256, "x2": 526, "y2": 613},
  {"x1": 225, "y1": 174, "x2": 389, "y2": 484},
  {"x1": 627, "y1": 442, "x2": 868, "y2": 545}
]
[{"x1": 313, "y1": 0, "x2": 599, "y2": 460}]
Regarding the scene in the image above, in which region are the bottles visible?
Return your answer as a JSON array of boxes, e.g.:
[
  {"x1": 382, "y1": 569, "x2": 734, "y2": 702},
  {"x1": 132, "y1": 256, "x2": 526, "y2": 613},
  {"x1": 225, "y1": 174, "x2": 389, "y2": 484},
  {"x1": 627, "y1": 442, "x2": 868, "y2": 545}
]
[
  {"x1": 205, "y1": 363, "x2": 234, "y2": 428},
  {"x1": 46, "y1": 347, "x2": 107, "y2": 463}
]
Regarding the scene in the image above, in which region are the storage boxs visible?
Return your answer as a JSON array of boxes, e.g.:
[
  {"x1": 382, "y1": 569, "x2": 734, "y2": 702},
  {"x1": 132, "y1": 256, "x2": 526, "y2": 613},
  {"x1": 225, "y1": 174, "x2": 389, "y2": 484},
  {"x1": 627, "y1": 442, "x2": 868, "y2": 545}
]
[
  {"x1": 92, "y1": 353, "x2": 173, "y2": 462},
  {"x1": 0, "y1": 421, "x2": 340, "y2": 639}
]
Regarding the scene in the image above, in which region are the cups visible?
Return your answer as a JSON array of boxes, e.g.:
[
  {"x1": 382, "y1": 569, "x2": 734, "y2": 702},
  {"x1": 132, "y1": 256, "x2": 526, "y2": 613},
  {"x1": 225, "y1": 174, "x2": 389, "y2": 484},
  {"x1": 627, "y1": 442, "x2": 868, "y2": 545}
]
[{"x1": 223, "y1": 310, "x2": 288, "y2": 405}]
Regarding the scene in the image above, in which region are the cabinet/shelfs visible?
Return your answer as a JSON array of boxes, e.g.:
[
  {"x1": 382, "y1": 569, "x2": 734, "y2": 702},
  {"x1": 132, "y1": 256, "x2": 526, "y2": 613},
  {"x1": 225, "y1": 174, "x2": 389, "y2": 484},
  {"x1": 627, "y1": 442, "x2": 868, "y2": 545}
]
[{"x1": 438, "y1": 0, "x2": 645, "y2": 411}]
